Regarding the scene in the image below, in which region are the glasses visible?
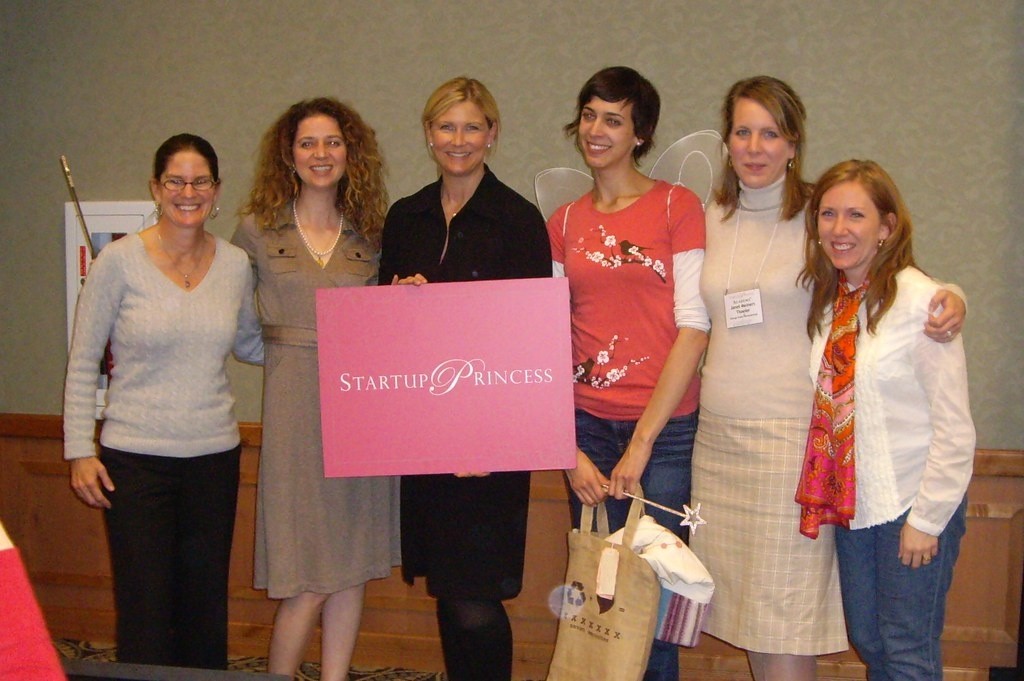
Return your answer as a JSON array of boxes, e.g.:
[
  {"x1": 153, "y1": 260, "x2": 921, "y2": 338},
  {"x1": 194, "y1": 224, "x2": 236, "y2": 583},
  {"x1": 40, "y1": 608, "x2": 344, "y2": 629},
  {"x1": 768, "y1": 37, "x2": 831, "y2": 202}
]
[{"x1": 155, "y1": 178, "x2": 218, "y2": 192}]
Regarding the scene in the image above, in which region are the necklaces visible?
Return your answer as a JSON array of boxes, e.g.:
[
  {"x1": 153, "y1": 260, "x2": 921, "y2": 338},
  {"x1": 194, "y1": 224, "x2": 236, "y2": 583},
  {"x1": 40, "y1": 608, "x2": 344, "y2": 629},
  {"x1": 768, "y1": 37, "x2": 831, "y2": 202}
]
[
  {"x1": 157, "y1": 221, "x2": 204, "y2": 288},
  {"x1": 293, "y1": 193, "x2": 344, "y2": 267},
  {"x1": 452, "y1": 212, "x2": 456, "y2": 218}
]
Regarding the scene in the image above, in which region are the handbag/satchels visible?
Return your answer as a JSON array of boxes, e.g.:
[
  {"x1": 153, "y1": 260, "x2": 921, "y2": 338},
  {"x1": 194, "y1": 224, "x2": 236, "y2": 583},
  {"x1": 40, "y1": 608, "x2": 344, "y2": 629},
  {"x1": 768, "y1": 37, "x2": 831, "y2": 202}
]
[{"x1": 545, "y1": 481, "x2": 662, "y2": 681}]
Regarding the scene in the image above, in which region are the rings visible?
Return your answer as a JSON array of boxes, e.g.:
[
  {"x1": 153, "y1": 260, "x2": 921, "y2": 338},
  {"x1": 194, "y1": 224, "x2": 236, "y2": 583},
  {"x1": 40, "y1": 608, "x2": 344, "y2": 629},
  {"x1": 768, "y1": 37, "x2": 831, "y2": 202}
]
[
  {"x1": 922, "y1": 555, "x2": 932, "y2": 561},
  {"x1": 947, "y1": 330, "x2": 954, "y2": 339}
]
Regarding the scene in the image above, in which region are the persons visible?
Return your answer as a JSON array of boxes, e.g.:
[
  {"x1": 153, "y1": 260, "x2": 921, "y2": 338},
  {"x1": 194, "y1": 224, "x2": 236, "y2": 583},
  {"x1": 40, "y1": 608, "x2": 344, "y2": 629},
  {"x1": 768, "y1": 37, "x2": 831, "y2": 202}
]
[
  {"x1": 796, "y1": 160, "x2": 976, "y2": 681},
  {"x1": 546, "y1": 67, "x2": 712, "y2": 681},
  {"x1": 227, "y1": 98, "x2": 400, "y2": 681},
  {"x1": 378, "y1": 75, "x2": 553, "y2": 681},
  {"x1": 62, "y1": 134, "x2": 266, "y2": 671},
  {"x1": 690, "y1": 75, "x2": 967, "y2": 681}
]
